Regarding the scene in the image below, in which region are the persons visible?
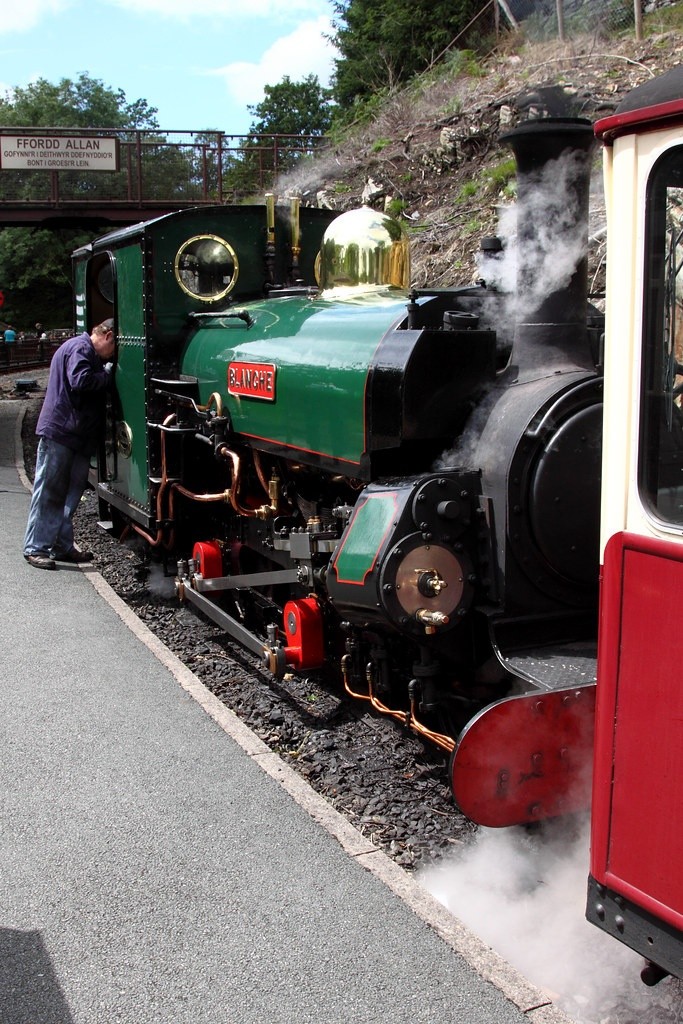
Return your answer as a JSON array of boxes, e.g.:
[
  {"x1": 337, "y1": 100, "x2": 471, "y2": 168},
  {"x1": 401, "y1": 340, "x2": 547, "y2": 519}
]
[
  {"x1": 4, "y1": 325, "x2": 16, "y2": 341},
  {"x1": 18, "y1": 318, "x2": 119, "y2": 568}
]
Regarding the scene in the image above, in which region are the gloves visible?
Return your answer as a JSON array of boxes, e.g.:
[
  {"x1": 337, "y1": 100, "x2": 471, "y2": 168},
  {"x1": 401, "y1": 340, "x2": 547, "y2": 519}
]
[{"x1": 105, "y1": 362, "x2": 113, "y2": 373}]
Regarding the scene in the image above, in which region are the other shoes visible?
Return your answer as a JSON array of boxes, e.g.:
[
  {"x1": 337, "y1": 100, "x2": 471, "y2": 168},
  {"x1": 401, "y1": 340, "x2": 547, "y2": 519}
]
[
  {"x1": 27, "y1": 555, "x2": 55, "y2": 568},
  {"x1": 50, "y1": 548, "x2": 94, "y2": 562}
]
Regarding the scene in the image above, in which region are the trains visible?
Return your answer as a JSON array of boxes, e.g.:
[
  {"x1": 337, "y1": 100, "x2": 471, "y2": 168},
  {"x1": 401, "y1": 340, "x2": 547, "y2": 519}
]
[{"x1": 71, "y1": 61, "x2": 683, "y2": 988}]
